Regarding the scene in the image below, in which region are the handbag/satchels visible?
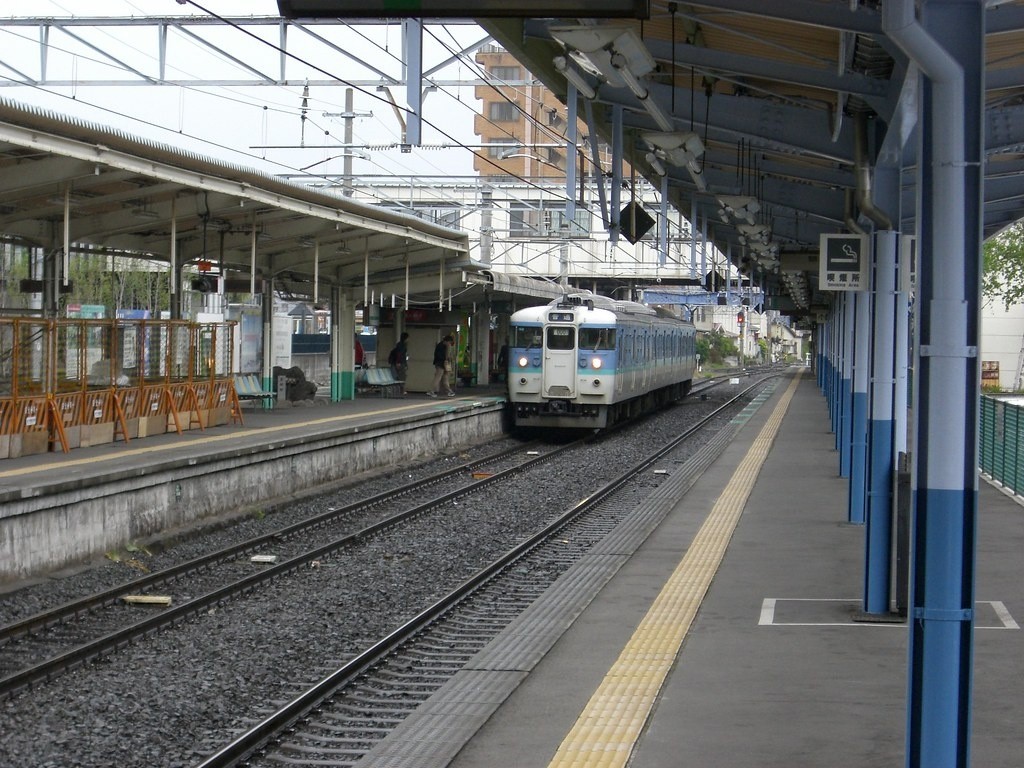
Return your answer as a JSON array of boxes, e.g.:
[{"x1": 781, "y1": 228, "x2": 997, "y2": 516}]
[{"x1": 444, "y1": 360, "x2": 453, "y2": 371}]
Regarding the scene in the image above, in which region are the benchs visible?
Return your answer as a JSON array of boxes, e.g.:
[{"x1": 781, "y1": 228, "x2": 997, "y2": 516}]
[{"x1": 457, "y1": 367, "x2": 475, "y2": 387}]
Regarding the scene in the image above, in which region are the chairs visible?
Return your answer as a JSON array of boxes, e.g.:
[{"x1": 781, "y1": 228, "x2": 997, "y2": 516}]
[
  {"x1": 233, "y1": 375, "x2": 278, "y2": 415},
  {"x1": 356, "y1": 366, "x2": 406, "y2": 399}
]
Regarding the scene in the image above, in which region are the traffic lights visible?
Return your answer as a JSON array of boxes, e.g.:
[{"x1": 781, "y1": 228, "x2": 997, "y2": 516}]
[{"x1": 737, "y1": 312, "x2": 744, "y2": 323}]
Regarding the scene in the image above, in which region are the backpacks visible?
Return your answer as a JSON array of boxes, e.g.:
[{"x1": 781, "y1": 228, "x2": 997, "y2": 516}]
[{"x1": 388, "y1": 348, "x2": 397, "y2": 364}]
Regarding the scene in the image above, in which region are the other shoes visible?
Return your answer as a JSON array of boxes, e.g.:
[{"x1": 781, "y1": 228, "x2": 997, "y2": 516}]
[
  {"x1": 425, "y1": 391, "x2": 438, "y2": 398},
  {"x1": 447, "y1": 392, "x2": 455, "y2": 396},
  {"x1": 402, "y1": 392, "x2": 408, "y2": 395}
]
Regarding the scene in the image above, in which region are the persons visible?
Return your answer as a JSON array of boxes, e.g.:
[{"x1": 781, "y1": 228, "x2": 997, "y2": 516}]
[
  {"x1": 388, "y1": 332, "x2": 409, "y2": 394},
  {"x1": 498, "y1": 336, "x2": 509, "y2": 395},
  {"x1": 426, "y1": 336, "x2": 455, "y2": 397},
  {"x1": 355, "y1": 334, "x2": 365, "y2": 393}
]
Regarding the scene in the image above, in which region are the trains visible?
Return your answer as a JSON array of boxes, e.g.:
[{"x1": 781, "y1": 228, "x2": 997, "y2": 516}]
[{"x1": 507, "y1": 289, "x2": 700, "y2": 438}]
